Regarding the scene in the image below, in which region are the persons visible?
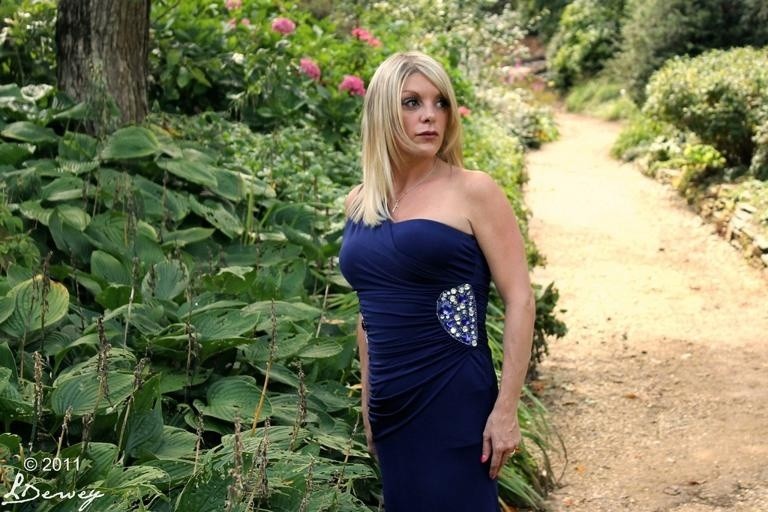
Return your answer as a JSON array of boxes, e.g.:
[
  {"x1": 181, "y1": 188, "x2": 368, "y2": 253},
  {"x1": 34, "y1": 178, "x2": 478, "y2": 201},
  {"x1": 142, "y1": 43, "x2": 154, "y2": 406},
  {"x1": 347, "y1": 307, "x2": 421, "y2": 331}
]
[{"x1": 339, "y1": 51, "x2": 536, "y2": 512}]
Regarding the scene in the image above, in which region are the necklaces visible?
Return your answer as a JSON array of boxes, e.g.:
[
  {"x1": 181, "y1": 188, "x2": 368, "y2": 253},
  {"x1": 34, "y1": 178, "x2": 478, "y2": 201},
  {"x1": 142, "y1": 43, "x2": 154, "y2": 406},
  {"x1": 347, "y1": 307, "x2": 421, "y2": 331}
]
[{"x1": 392, "y1": 161, "x2": 438, "y2": 213}]
